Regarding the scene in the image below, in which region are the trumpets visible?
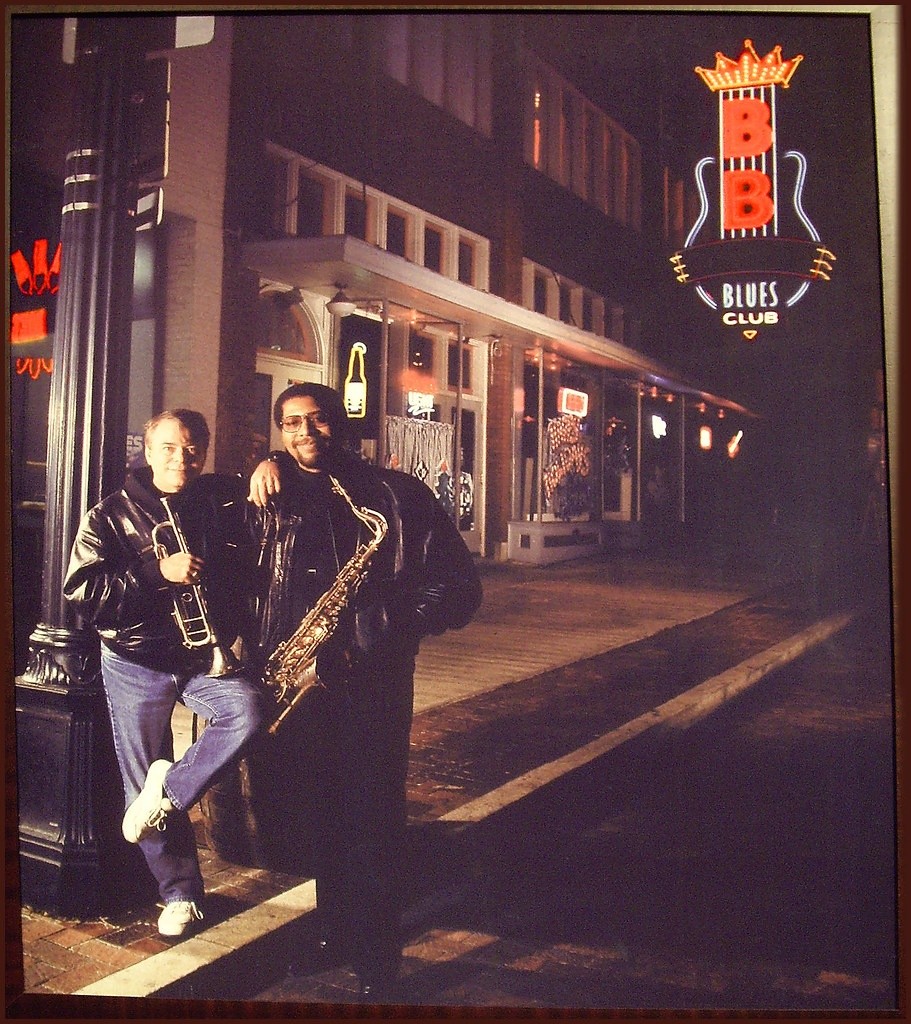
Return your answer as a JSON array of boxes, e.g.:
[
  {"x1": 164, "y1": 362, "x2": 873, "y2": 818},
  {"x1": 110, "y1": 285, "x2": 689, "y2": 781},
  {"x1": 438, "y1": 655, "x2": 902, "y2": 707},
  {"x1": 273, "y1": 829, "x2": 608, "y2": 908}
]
[{"x1": 151, "y1": 495, "x2": 250, "y2": 680}]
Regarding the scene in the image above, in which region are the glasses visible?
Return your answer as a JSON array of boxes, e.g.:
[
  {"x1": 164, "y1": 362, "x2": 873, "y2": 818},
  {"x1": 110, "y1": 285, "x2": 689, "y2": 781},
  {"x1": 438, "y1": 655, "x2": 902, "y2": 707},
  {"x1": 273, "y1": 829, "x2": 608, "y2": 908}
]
[{"x1": 280, "y1": 410, "x2": 330, "y2": 433}]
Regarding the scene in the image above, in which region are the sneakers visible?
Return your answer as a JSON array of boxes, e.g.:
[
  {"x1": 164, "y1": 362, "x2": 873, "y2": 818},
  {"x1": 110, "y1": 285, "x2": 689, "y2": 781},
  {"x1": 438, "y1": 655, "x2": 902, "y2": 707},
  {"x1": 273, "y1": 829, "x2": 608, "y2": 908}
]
[
  {"x1": 156, "y1": 901, "x2": 198, "y2": 936},
  {"x1": 122, "y1": 760, "x2": 177, "y2": 844}
]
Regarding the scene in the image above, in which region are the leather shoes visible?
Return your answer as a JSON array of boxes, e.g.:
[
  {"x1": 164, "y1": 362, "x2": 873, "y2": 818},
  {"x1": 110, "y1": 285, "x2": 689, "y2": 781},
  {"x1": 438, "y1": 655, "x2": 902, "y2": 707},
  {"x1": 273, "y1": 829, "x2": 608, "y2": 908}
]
[{"x1": 318, "y1": 931, "x2": 386, "y2": 997}]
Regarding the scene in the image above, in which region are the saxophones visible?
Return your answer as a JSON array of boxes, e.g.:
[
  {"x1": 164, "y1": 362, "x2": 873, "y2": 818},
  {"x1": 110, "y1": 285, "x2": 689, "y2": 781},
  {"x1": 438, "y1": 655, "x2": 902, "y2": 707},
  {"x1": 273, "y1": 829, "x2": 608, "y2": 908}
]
[{"x1": 260, "y1": 472, "x2": 387, "y2": 737}]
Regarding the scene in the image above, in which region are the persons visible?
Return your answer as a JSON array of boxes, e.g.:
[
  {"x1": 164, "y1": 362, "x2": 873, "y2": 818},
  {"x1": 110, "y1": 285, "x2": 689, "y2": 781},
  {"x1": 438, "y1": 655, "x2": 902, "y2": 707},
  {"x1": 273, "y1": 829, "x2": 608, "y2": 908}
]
[
  {"x1": 63, "y1": 407, "x2": 278, "y2": 945},
  {"x1": 234, "y1": 384, "x2": 485, "y2": 999}
]
